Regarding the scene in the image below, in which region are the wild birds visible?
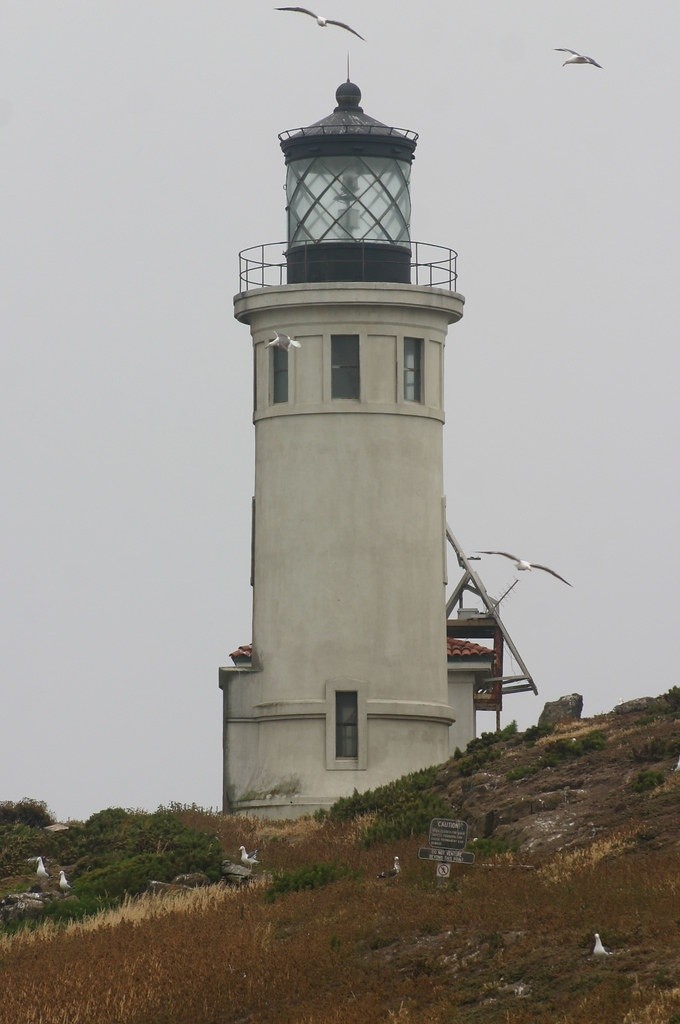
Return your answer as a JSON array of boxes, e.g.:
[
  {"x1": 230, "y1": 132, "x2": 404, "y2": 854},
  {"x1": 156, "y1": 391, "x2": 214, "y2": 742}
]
[
  {"x1": 554, "y1": 48, "x2": 606, "y2": 70},
  {"x1": 473, "y1": 551, "x2": 572, "y2": 587},
  {"x1": 36, "y1": 856, "x2": 51, "y2": 878},
  {"x1": 239, "y1": 846, "x2": 258, "y2": 870},
  {"x1": 59, "y1": 871, "x2": 72, "y2": 896},
  {"x1": 263, "y1": 331, "x2": 302, "y2": 351},
  {"x1": 273, "y1": 7, "x2": 367, "y2": 43},
  {"x1": 376, "y1": 857, "x2": 401, "y2": 879},
  {"x1": 593, "y1": 934, "x2": 613, "y2": 955}
]
